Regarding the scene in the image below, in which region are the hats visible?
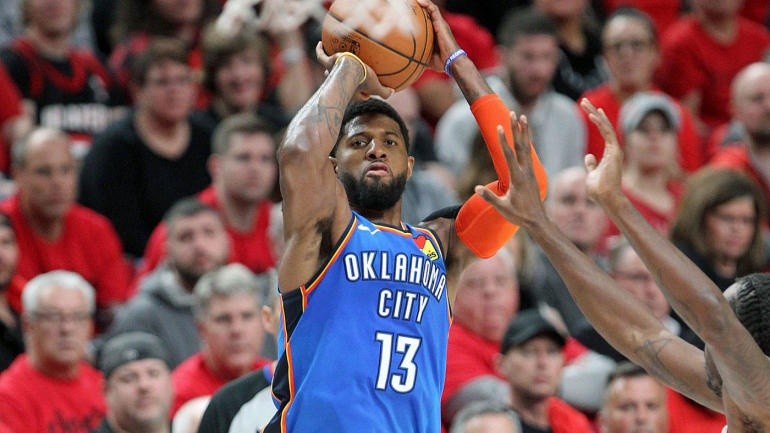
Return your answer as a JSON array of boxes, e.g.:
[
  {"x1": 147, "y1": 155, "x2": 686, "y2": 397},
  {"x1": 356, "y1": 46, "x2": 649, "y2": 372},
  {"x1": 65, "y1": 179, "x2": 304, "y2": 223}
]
[
  {"x1": 619, "y1": 90, "x2": 679, "y2": 135},
  {"x1": 500, "y1": 314, "x2": 566, "y2": 355},
  {"x1": 102, "y1": 328, "x2": 174, "y2": 379}
]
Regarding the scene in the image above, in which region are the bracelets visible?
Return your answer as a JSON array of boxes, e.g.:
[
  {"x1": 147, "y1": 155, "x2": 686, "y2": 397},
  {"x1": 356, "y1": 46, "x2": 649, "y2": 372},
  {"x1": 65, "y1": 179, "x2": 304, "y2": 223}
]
[
  {"x1": 444, "y1": 48, "x2": 468, "y2": 79},
  {"x1": 335, "y1": 51, "x2": 367, "y2": 85}
]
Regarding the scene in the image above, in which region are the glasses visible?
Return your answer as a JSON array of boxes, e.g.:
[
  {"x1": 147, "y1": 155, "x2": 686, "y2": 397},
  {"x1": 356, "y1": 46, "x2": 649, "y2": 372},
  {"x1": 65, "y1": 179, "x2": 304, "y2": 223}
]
[
  {"x1": 35, "y1": 311, "x2": 91, "y2": 324},
  {"x1": 604, "y1": 40, "x2": 655, "y2": 53}
]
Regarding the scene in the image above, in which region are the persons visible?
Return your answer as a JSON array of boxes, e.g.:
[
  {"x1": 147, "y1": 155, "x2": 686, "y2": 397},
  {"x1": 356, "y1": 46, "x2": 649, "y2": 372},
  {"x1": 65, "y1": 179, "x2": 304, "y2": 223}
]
[
  {"x1": 0, "y1": 0, "x2": 770, "y2": 432},
  {"x1": 264, "y1": 0, "x2": 545, "y2": 433},
  {"x1": 473, "y1": 95, "x2": 770, "y2": 433}
]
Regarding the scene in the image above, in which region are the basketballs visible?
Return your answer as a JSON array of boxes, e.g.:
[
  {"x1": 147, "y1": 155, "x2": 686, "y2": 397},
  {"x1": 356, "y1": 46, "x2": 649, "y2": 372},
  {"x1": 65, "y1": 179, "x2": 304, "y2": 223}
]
[{"x1": 321, "y1": 0, "x2": 437, "y2": 93}]
